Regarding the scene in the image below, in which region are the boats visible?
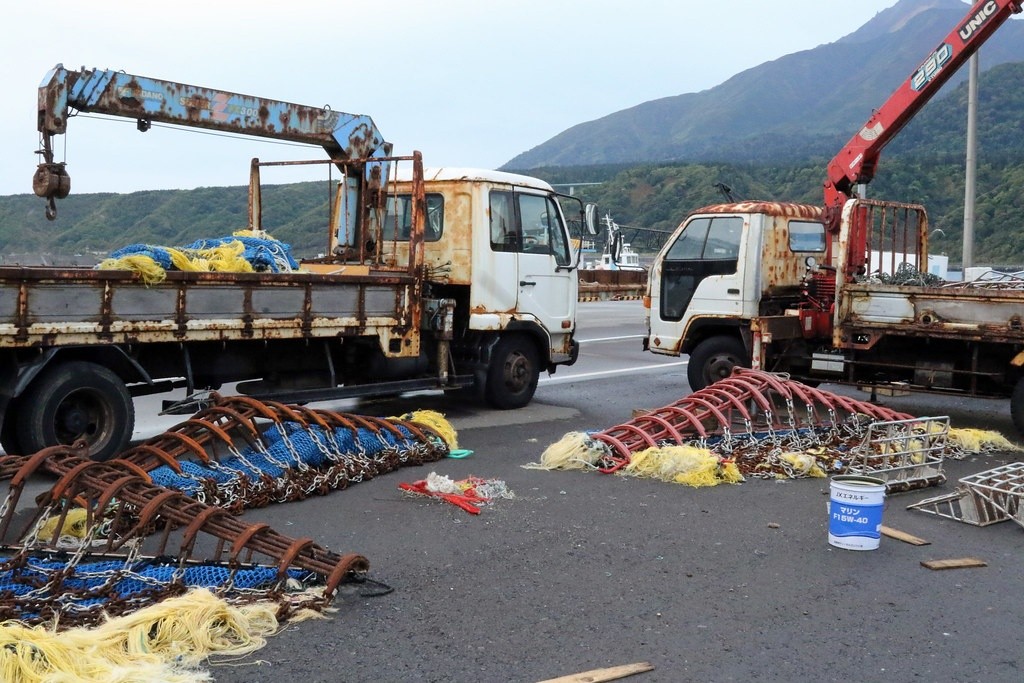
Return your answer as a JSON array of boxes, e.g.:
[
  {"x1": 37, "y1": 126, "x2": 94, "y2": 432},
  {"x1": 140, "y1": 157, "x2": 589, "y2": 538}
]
[{"x1": 587, "y1": 208, "x2": 645, "y2": 272}]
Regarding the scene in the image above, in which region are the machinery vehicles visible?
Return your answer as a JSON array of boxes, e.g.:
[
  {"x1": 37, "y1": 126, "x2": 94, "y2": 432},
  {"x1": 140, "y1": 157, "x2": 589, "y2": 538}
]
[
  {"x1": 0, "y1": 63, "x2": 603, "y2": 481},
  {"x1": 641, "y1": 0, "x2": 1023, "y2": 442}
]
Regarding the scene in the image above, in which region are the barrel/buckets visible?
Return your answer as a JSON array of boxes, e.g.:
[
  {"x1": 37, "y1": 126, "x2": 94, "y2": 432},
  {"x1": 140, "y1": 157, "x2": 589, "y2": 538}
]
[{"x1": 828, "y1": 474, "x2": 890, "y2": 551}]
